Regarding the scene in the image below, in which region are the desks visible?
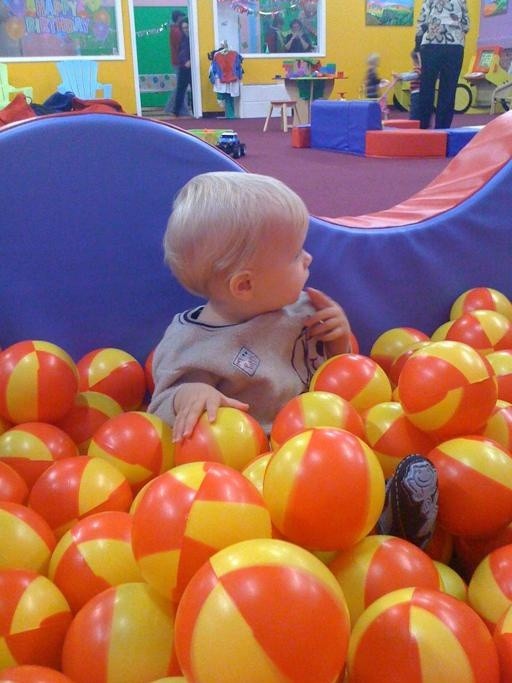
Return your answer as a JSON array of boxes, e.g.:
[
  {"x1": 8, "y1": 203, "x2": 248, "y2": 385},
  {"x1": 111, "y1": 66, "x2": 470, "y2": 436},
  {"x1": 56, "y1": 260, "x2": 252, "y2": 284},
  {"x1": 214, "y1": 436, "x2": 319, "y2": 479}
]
[{"x1": 274, "y1": 75, "x2": 336, "y2": 128}]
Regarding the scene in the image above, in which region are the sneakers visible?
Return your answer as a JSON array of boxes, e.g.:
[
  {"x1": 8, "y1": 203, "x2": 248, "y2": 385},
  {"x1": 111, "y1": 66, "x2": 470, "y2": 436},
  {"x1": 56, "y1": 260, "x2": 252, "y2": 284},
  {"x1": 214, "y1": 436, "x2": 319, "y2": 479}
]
[{"x1": 378, "y1": 454, "x2": 439, "y2": 550}]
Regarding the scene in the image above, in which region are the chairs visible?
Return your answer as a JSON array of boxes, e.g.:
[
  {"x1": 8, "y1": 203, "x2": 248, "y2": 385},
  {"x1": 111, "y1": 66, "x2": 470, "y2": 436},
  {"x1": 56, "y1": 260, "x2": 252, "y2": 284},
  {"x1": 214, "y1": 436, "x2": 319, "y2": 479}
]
[
  {"x1": 53, "y1": 58, "x2": 112, "y2": 100},
  {"x1": 0, "y1": 62, "x2": 32, "y2": 110}
]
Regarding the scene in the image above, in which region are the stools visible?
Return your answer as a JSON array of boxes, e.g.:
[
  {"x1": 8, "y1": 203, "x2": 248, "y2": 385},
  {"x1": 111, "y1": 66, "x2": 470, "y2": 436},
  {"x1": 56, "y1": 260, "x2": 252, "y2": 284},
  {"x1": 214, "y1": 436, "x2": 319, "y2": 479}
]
[{"x1": 263, "y1": 99, "x2": 302, "y2": 133}]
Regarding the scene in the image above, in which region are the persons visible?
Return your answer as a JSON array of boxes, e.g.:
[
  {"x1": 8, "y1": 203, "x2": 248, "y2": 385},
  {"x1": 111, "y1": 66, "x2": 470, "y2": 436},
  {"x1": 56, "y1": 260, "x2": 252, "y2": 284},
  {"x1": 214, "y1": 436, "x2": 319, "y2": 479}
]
[
  {"x1": 265, "y1": 16, "x2": 284, "y2": 52},
  {"x1": 164, "y1": 8, "x2": 186, "y2": 113},
  {"x1": 174, "y1": 16, "x2": 191, "y2": 117},
  {"x1": 415, "y1": 0, "x2": 471, "y2": 127},
  {"x1": 366, "y1": 53, "x2": 392, "y2": 99},
  {"x1": 147, "y1": 170, "x2": 440, "y2": 551},
  {"x1": 391, "y1": 45, "x2": 422, "y2": 119},
  {"x1": 284, "y1": 18, "x2": 311, "y2": 51}
]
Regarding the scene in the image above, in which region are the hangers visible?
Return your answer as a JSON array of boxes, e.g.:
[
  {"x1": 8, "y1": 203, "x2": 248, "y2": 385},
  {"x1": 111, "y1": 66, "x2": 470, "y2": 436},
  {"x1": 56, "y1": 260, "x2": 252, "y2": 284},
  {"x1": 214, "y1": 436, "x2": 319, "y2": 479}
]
[{"x1": 219, "y1": 44, "x2": 231, "y2": 54}]
